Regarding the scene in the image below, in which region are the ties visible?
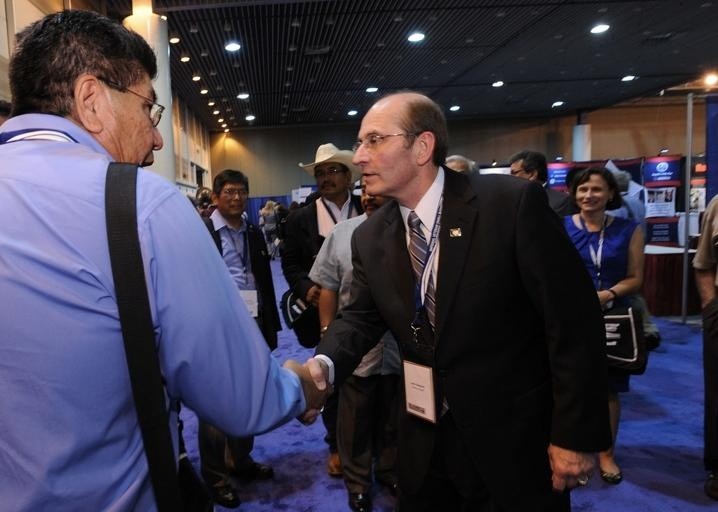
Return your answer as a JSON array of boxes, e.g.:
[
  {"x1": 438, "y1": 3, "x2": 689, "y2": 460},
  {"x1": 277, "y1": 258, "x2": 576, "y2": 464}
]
[{"x1": 408, "y1": 209, "x2": 438, "y2": 337}]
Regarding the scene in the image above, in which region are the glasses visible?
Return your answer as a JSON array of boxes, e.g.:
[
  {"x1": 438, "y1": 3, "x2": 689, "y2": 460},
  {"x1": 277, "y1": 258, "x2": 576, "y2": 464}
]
[
  {"x1": 510, "y1": 169, "x2": 524, "y2": 175},
  {"x1": 106, "y1": 83, "x2": 167, "y2": 129},
  {"x1": 352, "y1": 132, "x2": 420, "y2": 155},
  {"x1": 315, "y1": 169, "x2": 345, "y2": 180},
  {"x1": 220, "y1": 188, "x2": 249, "y2": 197}
]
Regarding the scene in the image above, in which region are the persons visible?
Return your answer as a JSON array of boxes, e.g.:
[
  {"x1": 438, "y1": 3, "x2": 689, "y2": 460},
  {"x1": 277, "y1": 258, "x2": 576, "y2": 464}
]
[
  {"x1": 279, "y1": 91, "x2": 612, "y2": 511},
  {"x1": 198, "y1": 169, "x2": 283, "y2": 508},
  {"x1": 0, "y1": 10, "x2": 335, "y2": 512},
  {"x1": 262, "y1": 143, "x2": 661, "y2": 485},
  {"x1": 692, "y1": 194, "x2": 718, "y2": 501}
]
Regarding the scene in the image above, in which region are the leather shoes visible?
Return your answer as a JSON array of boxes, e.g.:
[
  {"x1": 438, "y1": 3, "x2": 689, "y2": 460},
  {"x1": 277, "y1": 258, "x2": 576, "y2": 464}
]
[
  {"x1": 380, "y1": 478, "x2": 399, "y2": 499},
  {"x1": 600, "y1": 467, "x2": 624, "y2": 485},
  {"x1": 246, "y1": 462, "x2": 274, "y2": 480},
  {"x1": 329, "y1": 452, "x2": 344, "y2": 477},
  {"x1": 211, "y1": 483, "x2": 242, "y2": 509},
  {"x1": 348, "y1": 492, "x2": 373, "y2": 512},
  {"x1": 704, "y1": 471, "x2": 718, "y2": 501}
]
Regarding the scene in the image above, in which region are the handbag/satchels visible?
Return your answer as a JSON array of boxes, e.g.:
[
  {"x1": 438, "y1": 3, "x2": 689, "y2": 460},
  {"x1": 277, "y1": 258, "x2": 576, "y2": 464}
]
[
  {"x1": 604, "y1": 305, "x2": 650, "y2": 376},
  {"x1": 280, "y1": 288, "x2": 322, "y2": 350}
]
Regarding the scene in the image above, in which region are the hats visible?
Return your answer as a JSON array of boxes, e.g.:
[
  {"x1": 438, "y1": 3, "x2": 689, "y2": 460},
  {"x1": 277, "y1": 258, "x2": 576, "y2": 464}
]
[{"x1": 298, "y1": 143, "x2": 364, "y2": 186}]
